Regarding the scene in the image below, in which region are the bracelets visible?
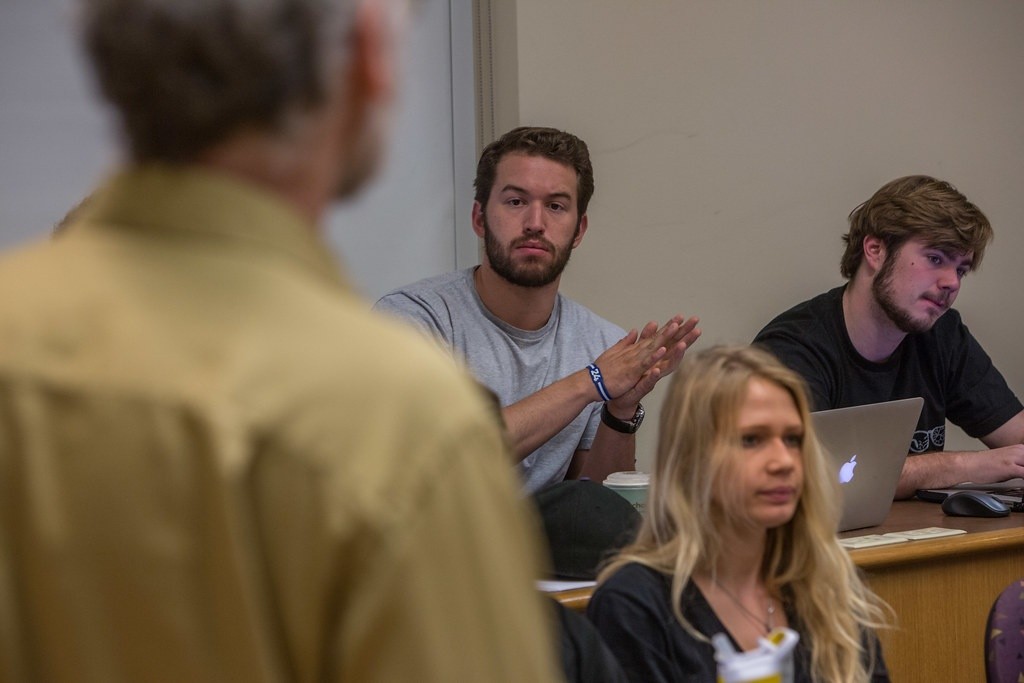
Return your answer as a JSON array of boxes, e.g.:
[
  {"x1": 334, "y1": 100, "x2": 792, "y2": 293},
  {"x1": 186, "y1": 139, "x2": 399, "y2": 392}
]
[{"x1": 586, "y1": 363, "x2": 613, "y2": 402}]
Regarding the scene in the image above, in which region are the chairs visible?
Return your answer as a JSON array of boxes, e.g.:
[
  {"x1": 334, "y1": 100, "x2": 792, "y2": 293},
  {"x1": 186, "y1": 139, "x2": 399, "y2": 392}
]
[{"x1": 983, "y1": 576, "x2": 1024, "y2": 683}]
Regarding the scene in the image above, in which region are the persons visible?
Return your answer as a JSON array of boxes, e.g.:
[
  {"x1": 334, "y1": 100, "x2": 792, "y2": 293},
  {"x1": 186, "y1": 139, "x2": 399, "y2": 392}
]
[
  {"x1": 0, "y1": 0, "x2": 568, "y2": 683},
  {"x1": 588, "y1": 341, "x2": 901, "y2": 683},
  {"x1": 373, "y1": 126, "x2": 702, "y2": 495},
  {"x1": 752, "y1": 174, "x2": 1024, "y2": 502},
  {"x1": 484, "y1": 389, "x2": 628, "y2": 683}
]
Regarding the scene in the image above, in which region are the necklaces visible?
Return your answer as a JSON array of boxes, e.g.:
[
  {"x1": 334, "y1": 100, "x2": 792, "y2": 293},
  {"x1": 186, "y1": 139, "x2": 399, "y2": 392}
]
[{"x1": 709, "y1": 573, "x2": 776, "y2": 633}]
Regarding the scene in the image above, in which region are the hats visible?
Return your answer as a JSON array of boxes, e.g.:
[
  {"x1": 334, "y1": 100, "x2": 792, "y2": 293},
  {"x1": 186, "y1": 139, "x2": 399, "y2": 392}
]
[{"x1": 527, "y1": 476, "x2": 643, "y2": 580}]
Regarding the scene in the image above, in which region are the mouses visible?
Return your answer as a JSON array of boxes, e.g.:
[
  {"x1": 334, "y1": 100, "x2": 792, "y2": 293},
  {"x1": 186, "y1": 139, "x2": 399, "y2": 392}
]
[{"x1": 941, "y1": 490, "x2": 1011, "y2": 518}]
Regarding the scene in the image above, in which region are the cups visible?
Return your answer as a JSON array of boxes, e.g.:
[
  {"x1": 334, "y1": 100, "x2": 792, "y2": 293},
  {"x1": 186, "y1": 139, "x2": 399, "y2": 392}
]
[
  {"x1": 709, "y1": 626, "x2": 800, "y2": 683},
  {"x1": 604, "y1": 470, "x2": 651, "y2": 517}
]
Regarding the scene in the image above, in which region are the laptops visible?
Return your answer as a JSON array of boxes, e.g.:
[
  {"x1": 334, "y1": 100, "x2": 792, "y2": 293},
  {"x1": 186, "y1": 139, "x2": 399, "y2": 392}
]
[
  {"x1": 808, "y1": 398, "x2": 925, "y2": 533},
  {"x1": 917, "y1": 475, "x2": 1024, "y2": 514}
]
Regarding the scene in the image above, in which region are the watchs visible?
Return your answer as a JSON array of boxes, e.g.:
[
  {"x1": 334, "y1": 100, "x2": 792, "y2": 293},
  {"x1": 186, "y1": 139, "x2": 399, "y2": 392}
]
[{"x1": 600, "y1": 401, "x2": 645, "y2": 434}]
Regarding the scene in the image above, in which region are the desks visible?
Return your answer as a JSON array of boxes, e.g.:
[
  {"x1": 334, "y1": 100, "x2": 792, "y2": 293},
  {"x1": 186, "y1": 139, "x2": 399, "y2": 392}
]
[{"x1": 543, "y1": 502, "x2": 1024, "y2": 683}]
